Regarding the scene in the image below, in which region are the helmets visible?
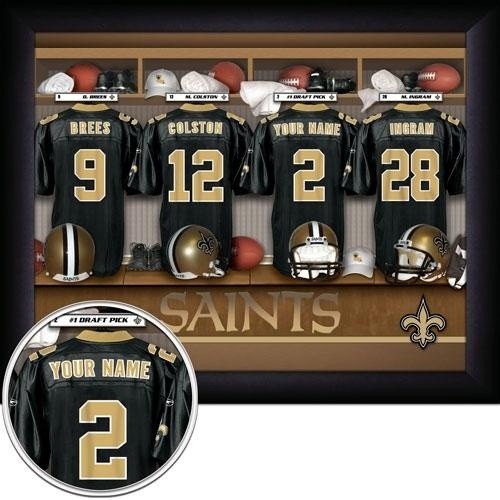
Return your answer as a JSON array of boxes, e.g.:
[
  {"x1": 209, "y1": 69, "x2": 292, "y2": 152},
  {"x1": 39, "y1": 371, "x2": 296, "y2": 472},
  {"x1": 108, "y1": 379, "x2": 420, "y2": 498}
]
[
  {"x1": 165, "y1": 224, "x2": 220, "y2": 281},
  {"x1": 286, "y1": 221, "x2": 340, "y2": 282},
  {"x1": 385, "y1": 224, "x2": 451, "y2": 286},
  {"x1": 44, "y1": 224, "x2": 95, "y2": 283}
]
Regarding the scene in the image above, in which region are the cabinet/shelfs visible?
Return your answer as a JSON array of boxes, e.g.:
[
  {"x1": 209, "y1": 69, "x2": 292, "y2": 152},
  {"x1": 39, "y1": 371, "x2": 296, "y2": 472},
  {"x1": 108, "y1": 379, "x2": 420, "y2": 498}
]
[{"x1": 33, "y1": 31, "x2": 468, "y2": 377}]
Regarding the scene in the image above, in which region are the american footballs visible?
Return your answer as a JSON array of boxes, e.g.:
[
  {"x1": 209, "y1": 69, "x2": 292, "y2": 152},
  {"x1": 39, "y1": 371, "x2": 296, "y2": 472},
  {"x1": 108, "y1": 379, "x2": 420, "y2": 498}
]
[
  {"x1": 34, "y1": 239, "x2": 46, "y2": 275},
  {"x1": 417, "y1": 63, "x2": 461, "y2": 93},
  {"x1": 279, "y1": 64, "x2": 314, "y2": 90},
  {"x1": 66, "y1": 63, "x2": 102, "y2": 92},
  {"x1": 209, "y1": 62, "x2": 244, "y2": 93},
  {"x1": 231, "y1": 236, "x2": 264, "y2": 270}
]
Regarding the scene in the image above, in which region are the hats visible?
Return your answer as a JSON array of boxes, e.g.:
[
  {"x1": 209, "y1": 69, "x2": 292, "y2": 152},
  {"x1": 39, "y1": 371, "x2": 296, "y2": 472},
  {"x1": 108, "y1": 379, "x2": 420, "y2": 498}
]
[
  {"x1": 340, "y1": 247, "x2": 377, "y2": 279},
  {"x1": 144, "y1": 68, "x2": 181, "y2": 99}
]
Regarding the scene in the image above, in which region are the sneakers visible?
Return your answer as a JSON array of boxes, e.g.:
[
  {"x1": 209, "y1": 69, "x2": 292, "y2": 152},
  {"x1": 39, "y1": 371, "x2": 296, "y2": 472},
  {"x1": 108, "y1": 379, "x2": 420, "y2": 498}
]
[
  {"x1": 307, "y1": 68, "x2": 327, "y2": 90},
  {"x1": 402, "y1": 72, "x2": 418, "y2": 91},
  {"x1": 149, "y1": 244, "x2": 162, "y2": 272},
  {"x1": 94, "y1": 71, "x2": 113, "y2": 92},
  {"x1": 326, "y1": 77, "x2": 356, "y2": 93},
  {"x1": 127, "y1": 243, "x2": 148, "y2": 271},
  {"x1": 108, "y1": 70, "x2": 136, "y2": 94}
]
